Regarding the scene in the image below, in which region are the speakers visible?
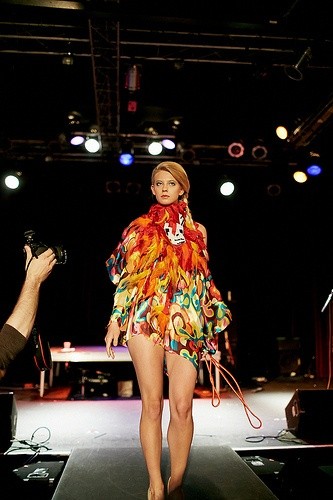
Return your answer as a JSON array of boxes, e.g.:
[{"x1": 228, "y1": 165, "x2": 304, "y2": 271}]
[
  {"x1": 0, "y1": 391, "x2": 19, "y2": 444},
  {"x1": 285, "y1": 388, "x2": 333, "y2": 445}
]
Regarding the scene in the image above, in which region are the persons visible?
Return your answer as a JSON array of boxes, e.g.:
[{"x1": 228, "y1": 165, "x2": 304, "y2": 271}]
[
  {"x1": 0, "y1": 240, "x2": 58, "y2": 380},
  {"x1": 99, "y1": 162, "x2": 235, "y2": 500}
]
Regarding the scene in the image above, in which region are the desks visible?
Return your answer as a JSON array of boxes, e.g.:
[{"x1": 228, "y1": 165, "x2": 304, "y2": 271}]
[{"x1": 37, "y1": 345, "x2": 220, "y2": 399}]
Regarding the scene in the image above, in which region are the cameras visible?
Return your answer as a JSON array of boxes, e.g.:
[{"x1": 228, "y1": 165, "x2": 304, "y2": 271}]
[{"x1": 24, "y1": 229, "x2": 68, "y2": 272}]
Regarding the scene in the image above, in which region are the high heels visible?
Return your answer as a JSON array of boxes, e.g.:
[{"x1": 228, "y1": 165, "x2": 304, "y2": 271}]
[
  {"x1": 167, "y1": 477, "x2": 184, "y2": 500},
  {"x1": 147, "y1": 483, "x2": 164, "y2": 500}
]
